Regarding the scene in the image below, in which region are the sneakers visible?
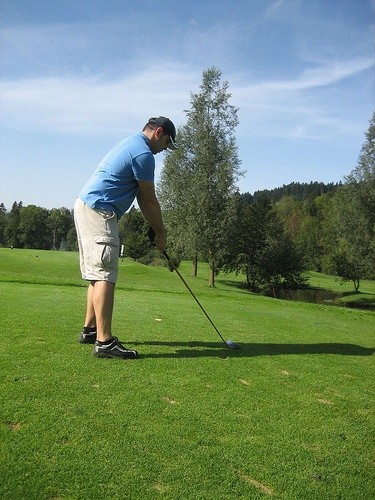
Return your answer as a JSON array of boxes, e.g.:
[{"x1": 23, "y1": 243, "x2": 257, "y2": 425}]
[
  {"x1": 94, "y1": 337, "x2": 138, "y2": 359},
  {"x1": 80, "y1": 327, "x2": 97, "y2": 344}
]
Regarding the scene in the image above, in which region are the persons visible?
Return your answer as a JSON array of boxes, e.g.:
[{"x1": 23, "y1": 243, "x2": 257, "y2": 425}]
[{"x1": 73, "y1": 115, "x2": 179, "y2": 360}]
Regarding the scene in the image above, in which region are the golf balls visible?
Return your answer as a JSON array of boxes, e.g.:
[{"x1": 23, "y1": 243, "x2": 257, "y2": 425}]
[{"x1": 227, "y1": 339, "x2": 232, "y2": 346}]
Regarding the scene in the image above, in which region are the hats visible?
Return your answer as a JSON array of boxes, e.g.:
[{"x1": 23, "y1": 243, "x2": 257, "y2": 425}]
[{"x1": 149, "y1": 115, "x2": 180, "y2": 151}]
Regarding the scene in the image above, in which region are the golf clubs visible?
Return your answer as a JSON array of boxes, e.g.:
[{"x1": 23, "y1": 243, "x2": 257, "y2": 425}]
[{"x1": 156, "y1": 240, "x2": 239, "y2": 349}]
